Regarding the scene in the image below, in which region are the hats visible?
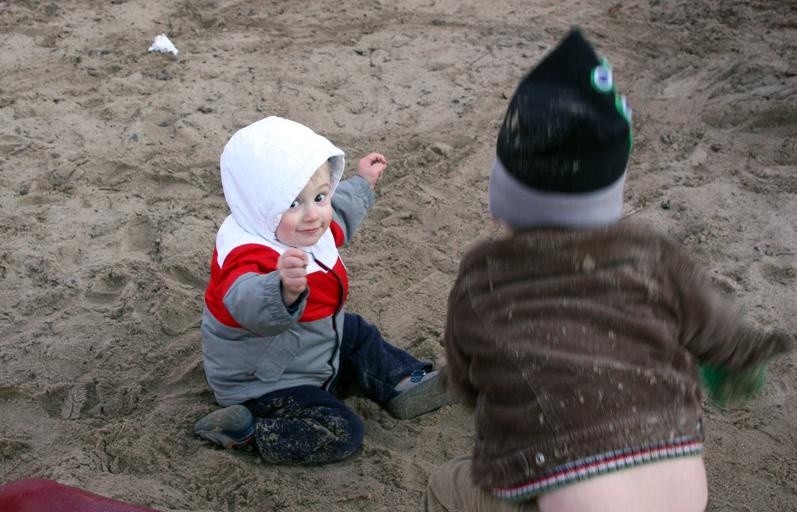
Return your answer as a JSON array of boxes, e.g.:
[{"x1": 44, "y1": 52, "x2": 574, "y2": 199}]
[{"x1": 486, "y1": 23, "x2": 634, "y2": 231}]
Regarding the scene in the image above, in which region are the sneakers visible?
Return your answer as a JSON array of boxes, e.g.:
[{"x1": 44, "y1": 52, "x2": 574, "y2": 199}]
[
  {"x1": 389, "y1": 367, "x2": 452, "y2": 421},
  {"x1": 192, "y1": 404, "x2": 253, "y2": 455}
]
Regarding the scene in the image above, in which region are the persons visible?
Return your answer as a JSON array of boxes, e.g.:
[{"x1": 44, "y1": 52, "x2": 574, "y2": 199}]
[
  {"x1": 190, "y1": 112, "x2": 466, "y2": 468},
  {"x1": 443, "y1": 77, "x2": 795, "y2": 510}
]
